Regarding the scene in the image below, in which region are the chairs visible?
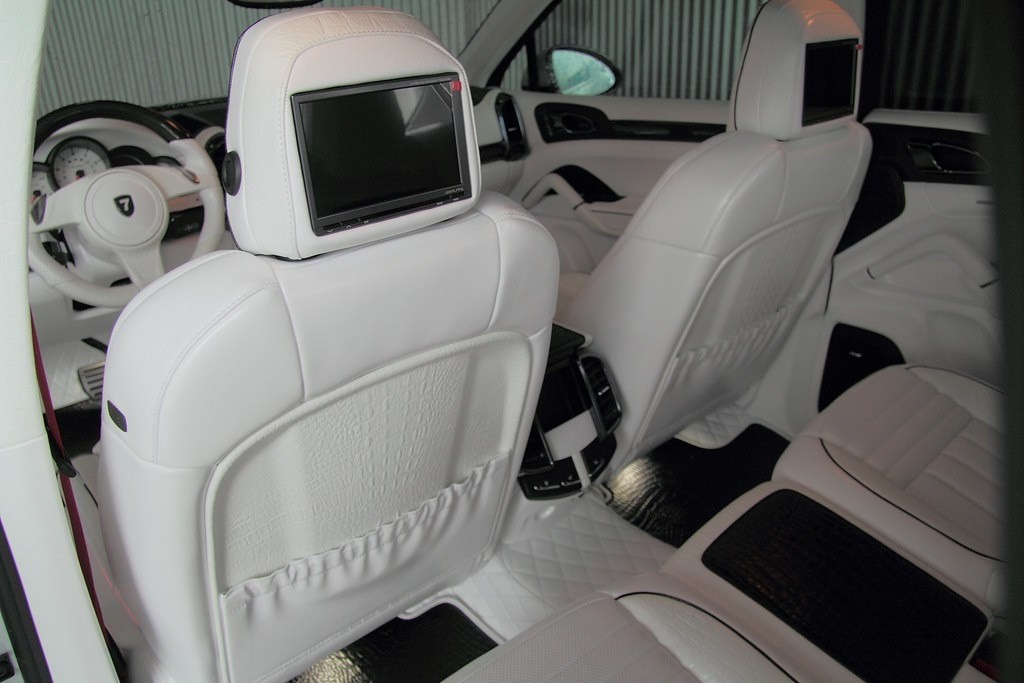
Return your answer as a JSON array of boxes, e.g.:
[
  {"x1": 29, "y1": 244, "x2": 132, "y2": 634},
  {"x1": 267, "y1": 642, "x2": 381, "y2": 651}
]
[
  {"x1": 552, "y1": 0, "x2": 874, "y2": 480},
  {"x1": 70, "y1": 8, "x2": 559, "y2": 683}
]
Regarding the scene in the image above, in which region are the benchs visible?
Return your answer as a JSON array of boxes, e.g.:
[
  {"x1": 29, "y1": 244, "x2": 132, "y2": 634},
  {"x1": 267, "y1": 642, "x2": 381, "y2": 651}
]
[{"x1": 445, "y1": 361, "x2": 1024, "y2": 683}]
